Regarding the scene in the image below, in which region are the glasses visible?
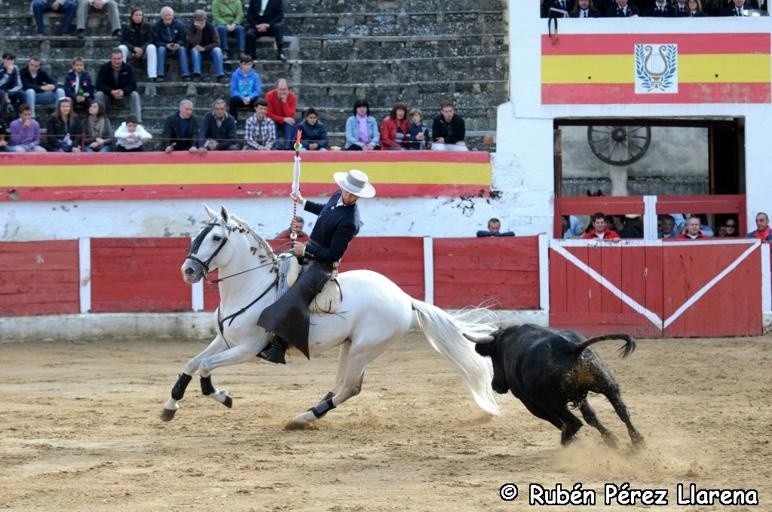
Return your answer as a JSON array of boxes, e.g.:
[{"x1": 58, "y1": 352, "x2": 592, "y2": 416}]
[{"x1": 726, "y1": 225, "x2": 736, "y2": 228}]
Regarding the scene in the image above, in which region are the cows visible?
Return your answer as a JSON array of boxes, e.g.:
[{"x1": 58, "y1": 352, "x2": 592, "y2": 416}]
[{"x1": 463, "y1": 322, "x2": 646, "y2": 454}]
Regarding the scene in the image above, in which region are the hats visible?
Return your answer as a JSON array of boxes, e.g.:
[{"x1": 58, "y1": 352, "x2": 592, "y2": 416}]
[{"x1": 334, "y1": 170, "x2": 376, "y2": 198}]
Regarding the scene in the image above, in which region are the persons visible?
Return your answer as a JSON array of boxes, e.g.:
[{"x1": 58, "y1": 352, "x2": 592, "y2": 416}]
[
  {"x1": 476, "y1": 218, "x2": 517, "y2": 237},
  {"x1": 293, "y1": 107, "x2": 331, "y2": 153},
  {"x1": 604, "y1": 0, "x2": 640, "y2": 16},
  {"x1": 431, "y1": 101, "x2": 469, "y2": 152},
  {"x1": 559, "y1": 213, "x2": 738, "y2": 242},
  {"x1": 343, "y1": 99, "x2": 382, "y2": 150},
  {"x1": 543, "y1": 0, "x2": 576, "y2": 18},
  {"x1": 673, "y1": 0, "x2": 690, "y2": 16},
  {"x1": 683, "y1": 0, "x2": 708, "y2": 17},
  {"x1": 403, "y1": 107, "x2": 430, "y2": 149},
  {"x1": 643, "y1": 0, "x2": 677, "y2": 16},
  {"x1": 562, "y1": 1, "x2": 603, "y2": 18},
  {"x1": 273, "y1": 215, "x2": 309, "y2": 239},
  {"x1": 0, "y1": 1, "x2": 299, "y2": 154},
  {"x1": 379, "y1": 101, "x2": 410, "y2": 150},
  {"x1": 724, "y1": 0, "x2": 754, "y2": 17},
  {"x1": 746, "y1": 211, "x2": 771, "y2": 289},
  {"x1": 254, "y1": 167, "x2": 376, "y2": 367}
]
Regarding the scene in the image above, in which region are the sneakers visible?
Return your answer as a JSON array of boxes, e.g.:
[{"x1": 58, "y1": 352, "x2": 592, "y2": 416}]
[
  {"x1": 184, "y1": 77, "x2": 225, "y2": 82},
  {"x1": 151, "y1": 77, "x2": 164, "y2": 81},
  {"x1": 223, "y1": 53, "x2": 244, "y2": 60},
  {"x1": 278, "y1": 53, "x2": 286, "y2": 61}
]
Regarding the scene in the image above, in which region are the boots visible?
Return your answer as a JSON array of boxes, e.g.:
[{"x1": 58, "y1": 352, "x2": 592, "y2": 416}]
[{"x1": 261, "y1": 335, "x2": 289, "y2": 360}]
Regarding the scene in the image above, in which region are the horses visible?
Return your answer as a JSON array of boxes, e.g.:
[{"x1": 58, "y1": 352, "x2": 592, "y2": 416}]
[{"x1": 158, "y1": 202, "x2": 502, "y2": 431}]
